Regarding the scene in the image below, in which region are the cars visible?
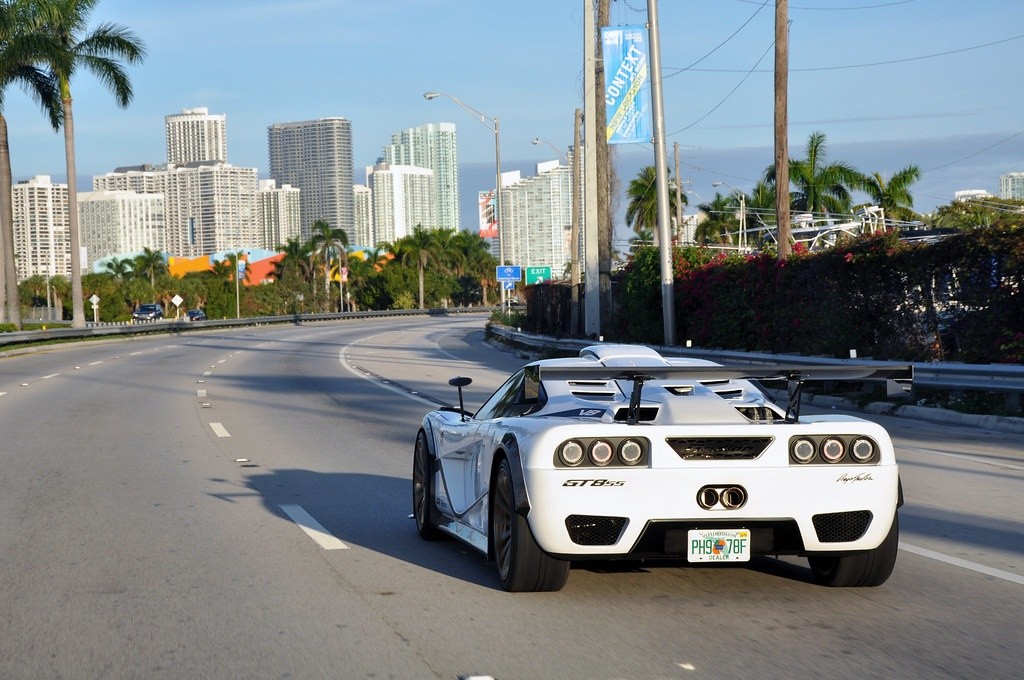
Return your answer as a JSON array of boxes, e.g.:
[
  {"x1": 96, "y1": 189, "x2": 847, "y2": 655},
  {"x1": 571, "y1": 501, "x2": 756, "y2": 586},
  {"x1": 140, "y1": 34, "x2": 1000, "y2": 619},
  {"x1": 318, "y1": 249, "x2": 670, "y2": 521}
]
[{"x1": 187, "y1": 309, "x2": 207, "y2": 321}]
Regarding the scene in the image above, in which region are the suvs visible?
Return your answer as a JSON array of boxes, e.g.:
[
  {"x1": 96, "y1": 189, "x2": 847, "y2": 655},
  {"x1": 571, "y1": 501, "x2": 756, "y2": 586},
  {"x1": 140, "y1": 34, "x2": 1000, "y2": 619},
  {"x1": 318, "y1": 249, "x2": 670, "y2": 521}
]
[{"x1": 132, "y1": 304, "x2": 165, "y2": 323}]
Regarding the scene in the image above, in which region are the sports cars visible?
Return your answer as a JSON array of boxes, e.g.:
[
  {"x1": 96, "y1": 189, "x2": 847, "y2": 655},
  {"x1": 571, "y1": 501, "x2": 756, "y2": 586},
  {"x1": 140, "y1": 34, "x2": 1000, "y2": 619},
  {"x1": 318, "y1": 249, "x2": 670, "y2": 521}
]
[{"x1": 411, "y1": 341, "x2": 905, "y2": 595}]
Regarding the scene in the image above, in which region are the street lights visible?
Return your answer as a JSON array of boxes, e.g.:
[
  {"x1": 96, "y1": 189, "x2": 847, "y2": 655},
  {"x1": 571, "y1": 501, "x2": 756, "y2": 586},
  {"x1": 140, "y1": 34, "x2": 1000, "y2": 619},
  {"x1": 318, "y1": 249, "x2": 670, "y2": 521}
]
[
  {"x1": 711, "y1": 181, "x2": 748, "y2": 249},
  {"x1": 533, "y1": 138, "x2": 580, "y2": 333},
  {"x1": 237, "y1": 208, "x2": 250, "y2": 319},
  {"x1": 423, "y1": 90, "x2": 505, "y2": 315}
]
